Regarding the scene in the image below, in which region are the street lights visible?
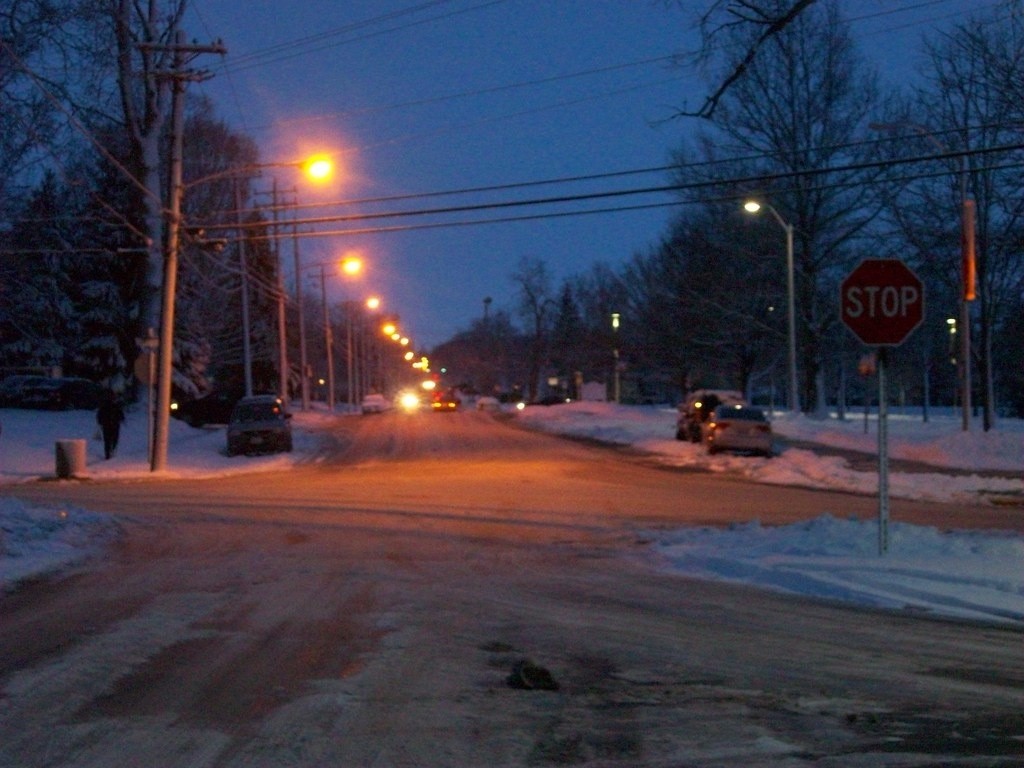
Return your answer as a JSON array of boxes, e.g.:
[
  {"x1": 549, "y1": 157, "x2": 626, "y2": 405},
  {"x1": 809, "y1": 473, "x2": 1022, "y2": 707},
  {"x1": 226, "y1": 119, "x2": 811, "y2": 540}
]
[
  {"x1": 277, "y1": 255, "x2": 365, "y2": 399},
  {"x1": 146, "y1": 153, "x2": 336, "y2": 472},
  {"x1": 346, "y1": 297, "x2": 414, "y2": 409},
  {"x1": 742, "y1": 199, "x2": 803, "y2": 416}
]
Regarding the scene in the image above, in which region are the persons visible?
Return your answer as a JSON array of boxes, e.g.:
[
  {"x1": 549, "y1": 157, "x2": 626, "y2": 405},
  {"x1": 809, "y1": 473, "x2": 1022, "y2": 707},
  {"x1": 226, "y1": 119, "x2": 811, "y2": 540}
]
[{"x1": 95, "y1": 390, "x2": 126, "y2": 460}]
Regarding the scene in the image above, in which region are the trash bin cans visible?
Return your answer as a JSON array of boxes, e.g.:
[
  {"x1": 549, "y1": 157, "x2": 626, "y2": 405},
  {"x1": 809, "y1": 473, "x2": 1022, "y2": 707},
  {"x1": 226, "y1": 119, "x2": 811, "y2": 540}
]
[{"x1": 54, "y1": 437, "x2": 87, "y2": 478}]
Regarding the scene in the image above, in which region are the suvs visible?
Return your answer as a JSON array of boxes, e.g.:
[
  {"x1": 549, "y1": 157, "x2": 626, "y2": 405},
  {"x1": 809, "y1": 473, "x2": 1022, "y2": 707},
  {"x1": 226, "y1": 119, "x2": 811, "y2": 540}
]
[{"x1": 225, "y1": 394, "x2": 295, "y2": 458}]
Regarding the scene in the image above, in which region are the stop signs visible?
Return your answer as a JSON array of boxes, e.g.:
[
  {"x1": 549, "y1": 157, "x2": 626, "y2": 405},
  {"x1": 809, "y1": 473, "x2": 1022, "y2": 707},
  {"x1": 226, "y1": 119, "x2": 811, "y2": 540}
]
[{"x1": 838, "y1": 257, "x2": 927, "y2": 348}]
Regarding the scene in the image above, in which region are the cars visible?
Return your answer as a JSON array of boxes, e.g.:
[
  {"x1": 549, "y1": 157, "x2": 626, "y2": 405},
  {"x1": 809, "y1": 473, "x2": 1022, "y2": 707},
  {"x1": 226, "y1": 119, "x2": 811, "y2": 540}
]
[
  {"x1": 430, "y1": 393, "x2": 456, "y2": 411},
  {"x1": 699, "y1": 401, "x2": 772, "y2": 458},
  {"x1": 361, "y1": 392, "x2": 385, "y2": 414},
  {"x1": 517, "y1": 394, "x2": 571, "y2": 408},
  {"x1": 673, "y1": 389, "x2": 746, "y2": 443}
]
